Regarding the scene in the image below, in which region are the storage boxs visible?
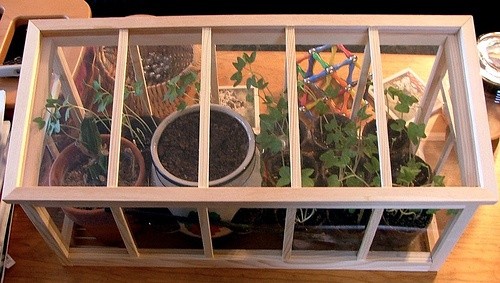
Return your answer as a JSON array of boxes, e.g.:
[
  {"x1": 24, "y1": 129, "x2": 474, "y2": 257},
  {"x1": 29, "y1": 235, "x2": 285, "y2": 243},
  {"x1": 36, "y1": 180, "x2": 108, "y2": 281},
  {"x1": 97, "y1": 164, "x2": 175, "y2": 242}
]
[{"x1": 1, "y1": 13, "x2": 500, "y2": 272}]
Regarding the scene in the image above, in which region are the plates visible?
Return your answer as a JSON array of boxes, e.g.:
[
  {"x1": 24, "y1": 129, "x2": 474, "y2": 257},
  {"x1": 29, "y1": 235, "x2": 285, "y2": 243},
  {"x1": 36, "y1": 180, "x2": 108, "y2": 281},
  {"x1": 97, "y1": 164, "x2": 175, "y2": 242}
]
[{"x1": 477, "y1": 32, "x2": 500, "y2": 87}]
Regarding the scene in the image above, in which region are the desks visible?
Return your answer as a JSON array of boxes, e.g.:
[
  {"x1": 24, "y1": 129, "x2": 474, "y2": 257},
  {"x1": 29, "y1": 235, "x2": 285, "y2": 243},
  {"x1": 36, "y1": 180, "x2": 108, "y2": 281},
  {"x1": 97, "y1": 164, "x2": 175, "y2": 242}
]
[{"x1": 1, "y1": 50, "x2": 499, "y2": 283}]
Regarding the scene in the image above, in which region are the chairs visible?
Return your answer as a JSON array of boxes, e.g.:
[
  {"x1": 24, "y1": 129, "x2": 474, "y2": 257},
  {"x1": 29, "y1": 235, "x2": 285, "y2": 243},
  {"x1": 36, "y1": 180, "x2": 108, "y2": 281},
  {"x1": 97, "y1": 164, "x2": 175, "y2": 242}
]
[{"x1": 0, "y1": 0, "x2": 92, "y2": 109}]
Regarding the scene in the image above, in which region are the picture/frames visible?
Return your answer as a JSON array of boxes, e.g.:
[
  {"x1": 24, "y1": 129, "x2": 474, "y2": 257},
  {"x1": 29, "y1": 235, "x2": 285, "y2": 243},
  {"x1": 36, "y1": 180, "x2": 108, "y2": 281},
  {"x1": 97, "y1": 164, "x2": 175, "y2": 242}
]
[{"x1": 368, "y1": 67, "x2": 445, "y2": 128}]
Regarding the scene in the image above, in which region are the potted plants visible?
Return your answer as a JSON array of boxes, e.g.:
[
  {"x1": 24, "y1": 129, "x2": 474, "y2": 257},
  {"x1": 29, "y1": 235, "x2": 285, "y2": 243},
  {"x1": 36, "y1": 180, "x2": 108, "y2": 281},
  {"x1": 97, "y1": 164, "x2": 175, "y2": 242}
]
[{"x1": 32, "y1": 52, "x2": 462, "y2": 238}]
